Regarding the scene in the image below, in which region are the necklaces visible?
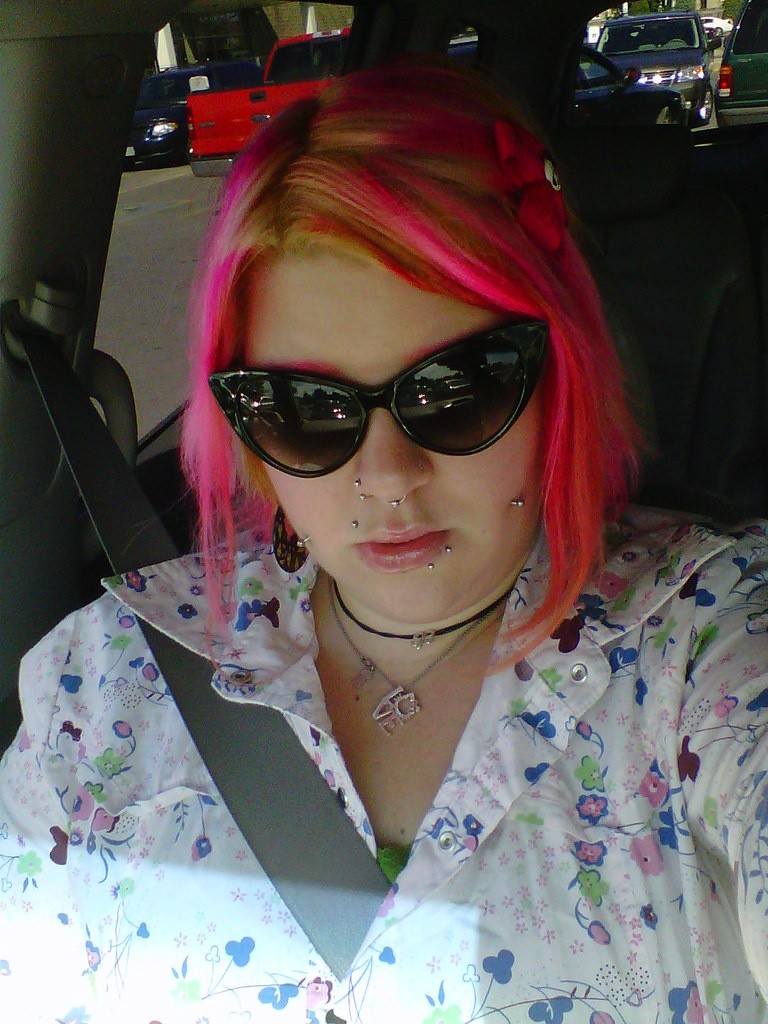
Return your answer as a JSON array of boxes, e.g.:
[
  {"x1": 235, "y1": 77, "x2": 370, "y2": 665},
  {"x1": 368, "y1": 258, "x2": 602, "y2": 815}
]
[{"x1": 327, "y1": 578, "x2": 512, "y2": 737}]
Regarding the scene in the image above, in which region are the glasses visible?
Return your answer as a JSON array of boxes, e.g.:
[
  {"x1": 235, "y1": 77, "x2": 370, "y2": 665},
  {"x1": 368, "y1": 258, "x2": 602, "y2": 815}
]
[{"x1": 207, "y1": 315, "x2": 551, "y2": 479}]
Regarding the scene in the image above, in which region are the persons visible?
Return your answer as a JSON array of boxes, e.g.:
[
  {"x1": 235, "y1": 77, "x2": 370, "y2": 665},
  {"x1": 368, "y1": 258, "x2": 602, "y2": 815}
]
[{"x1": 0, "y1": 68, "x2": 768, "y2": 1023}]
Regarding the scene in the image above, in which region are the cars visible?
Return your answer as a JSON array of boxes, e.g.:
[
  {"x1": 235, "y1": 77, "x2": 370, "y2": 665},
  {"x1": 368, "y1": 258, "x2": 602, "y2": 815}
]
[
  {"x1": 444, "y1": 35, "x2": 690, "y2": 128},
  {"x1": 700, "y1": 16, "x2": 734, "y2": 37}
]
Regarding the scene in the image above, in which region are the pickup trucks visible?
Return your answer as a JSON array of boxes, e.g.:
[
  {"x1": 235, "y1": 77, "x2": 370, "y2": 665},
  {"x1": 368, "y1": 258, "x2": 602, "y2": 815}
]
[{"x1": 186, "y1": 27, "x2": 355, "y2": 183}]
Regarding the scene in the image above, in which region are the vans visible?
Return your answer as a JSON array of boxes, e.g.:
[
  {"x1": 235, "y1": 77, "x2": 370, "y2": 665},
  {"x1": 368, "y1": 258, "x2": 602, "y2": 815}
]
[
  {"x1": 588, "y1": 11, "x2": 722, "y2": 128},
  {"x1": 714, "y1": 0, "x2": 767, "y2": 130},
  {"x1": 127, "y1": 59, "x2": 265, "y2": 172}
]
